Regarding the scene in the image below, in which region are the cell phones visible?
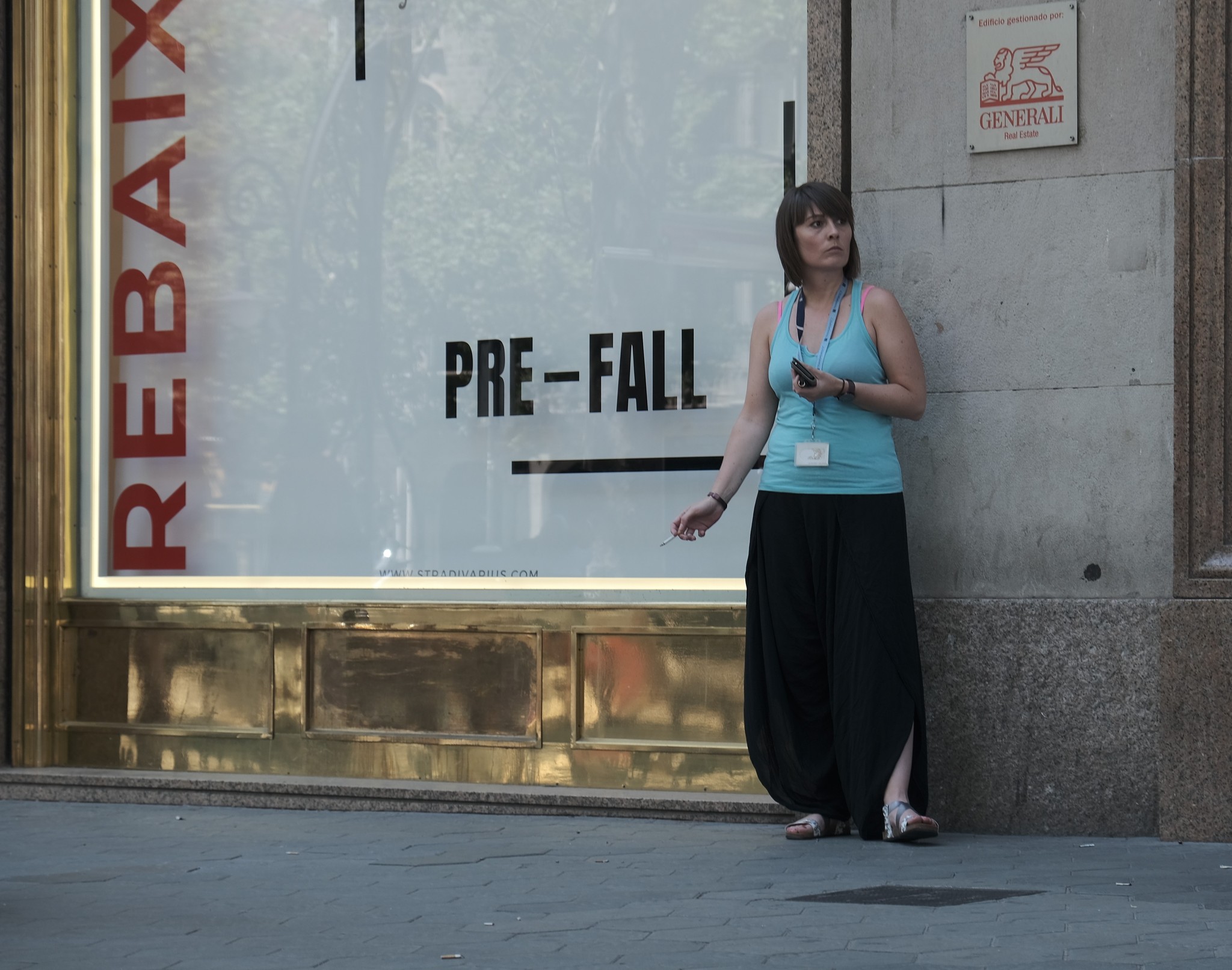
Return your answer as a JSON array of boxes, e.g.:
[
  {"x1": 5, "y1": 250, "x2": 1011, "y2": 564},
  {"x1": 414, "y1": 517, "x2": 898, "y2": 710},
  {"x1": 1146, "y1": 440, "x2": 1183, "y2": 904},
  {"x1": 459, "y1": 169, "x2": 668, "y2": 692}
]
[{"x1": 791, "y1": 357, "x2": 818, "y2": 386}]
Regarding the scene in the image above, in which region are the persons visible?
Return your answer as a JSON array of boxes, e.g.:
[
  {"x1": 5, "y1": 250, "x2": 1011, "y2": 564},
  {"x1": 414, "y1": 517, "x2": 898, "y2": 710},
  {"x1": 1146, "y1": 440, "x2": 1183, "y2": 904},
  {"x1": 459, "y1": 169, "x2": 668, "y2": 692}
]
[{"x1": 671, "y1": 180, "x2": 940, "y2": 841}]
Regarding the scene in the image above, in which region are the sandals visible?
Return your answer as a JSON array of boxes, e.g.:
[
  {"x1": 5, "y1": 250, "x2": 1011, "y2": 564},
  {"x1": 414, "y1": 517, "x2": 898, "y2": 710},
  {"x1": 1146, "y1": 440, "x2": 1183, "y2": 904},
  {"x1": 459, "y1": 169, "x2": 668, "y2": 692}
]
[
  {"x1": 784, "y1": 812, "x2": 851, "y2": 839},
  {"x1": 882, "y1": 800, "x2": 940, "y2": 841}
]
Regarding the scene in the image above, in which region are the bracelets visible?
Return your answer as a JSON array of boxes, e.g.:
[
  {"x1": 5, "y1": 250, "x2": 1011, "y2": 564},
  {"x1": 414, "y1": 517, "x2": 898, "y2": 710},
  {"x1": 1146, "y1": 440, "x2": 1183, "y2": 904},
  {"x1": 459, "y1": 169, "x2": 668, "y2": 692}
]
[
  {"x1": 833, "y1": 378, "x2": 845, "y2": 398},
  {"x1": 707, "y1": 492, "x2": 728, "y2": 511}
]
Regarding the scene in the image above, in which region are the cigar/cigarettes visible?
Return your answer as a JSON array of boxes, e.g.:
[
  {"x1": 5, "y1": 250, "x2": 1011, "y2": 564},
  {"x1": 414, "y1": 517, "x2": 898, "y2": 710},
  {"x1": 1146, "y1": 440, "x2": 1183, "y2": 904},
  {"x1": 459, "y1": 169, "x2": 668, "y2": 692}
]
[{"x1": 659, "y1": 535, "x2": 674, "y2": 547}]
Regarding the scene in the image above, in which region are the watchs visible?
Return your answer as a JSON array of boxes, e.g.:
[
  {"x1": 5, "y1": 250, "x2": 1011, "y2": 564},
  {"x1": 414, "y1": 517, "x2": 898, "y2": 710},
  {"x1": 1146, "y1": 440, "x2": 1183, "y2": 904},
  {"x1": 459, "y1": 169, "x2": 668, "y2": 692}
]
[{"x1": 838, "y1": 378, "x2": 855, "y2": 403}]
